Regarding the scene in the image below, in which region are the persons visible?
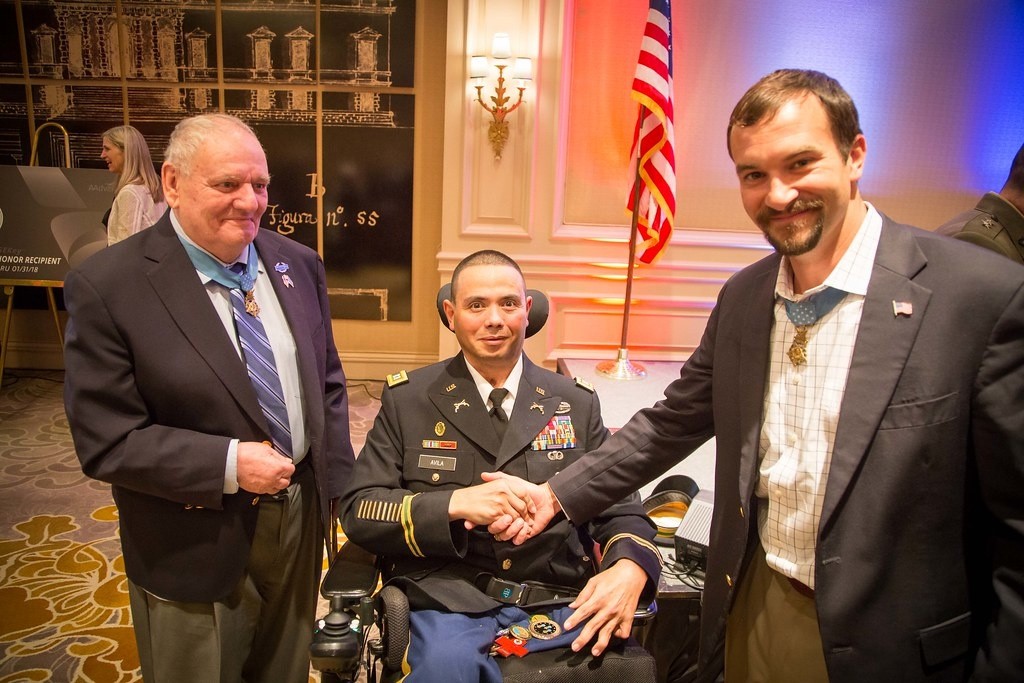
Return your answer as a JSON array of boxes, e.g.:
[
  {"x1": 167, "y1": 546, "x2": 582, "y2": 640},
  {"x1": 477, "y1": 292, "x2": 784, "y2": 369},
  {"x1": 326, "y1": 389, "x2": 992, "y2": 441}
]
[
  {"x1": 338, "y1": 250, "x2": 662, "y2": 683},
  {"x1": 934, "y1": 137, "x2": 1024, "y2": 265},
  {"x1": 63, "y1": 114, "x2": 356, "y2": 683},
  {"x1": 95, "y1": 127, "x2": 171, "y2": 246},
  {"x1": 481, "y1": 70, "x2": 1023, "y2": 683}
]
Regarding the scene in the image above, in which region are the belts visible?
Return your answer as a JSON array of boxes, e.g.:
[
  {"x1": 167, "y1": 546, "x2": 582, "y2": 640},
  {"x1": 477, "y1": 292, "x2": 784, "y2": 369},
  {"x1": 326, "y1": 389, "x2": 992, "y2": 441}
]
[{"x1": 292, "y1": 456, "x2": 311, "y2": 475}]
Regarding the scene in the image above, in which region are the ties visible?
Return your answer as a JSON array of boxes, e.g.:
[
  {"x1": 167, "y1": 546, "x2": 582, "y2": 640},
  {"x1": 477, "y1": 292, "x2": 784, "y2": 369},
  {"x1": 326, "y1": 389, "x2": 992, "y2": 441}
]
[
  {"x1": 227, "y1": 264, "x2": 295, "y2": 463},
  {"x1": 488, "y1": 388, "x2": 509, "y2": 444}
]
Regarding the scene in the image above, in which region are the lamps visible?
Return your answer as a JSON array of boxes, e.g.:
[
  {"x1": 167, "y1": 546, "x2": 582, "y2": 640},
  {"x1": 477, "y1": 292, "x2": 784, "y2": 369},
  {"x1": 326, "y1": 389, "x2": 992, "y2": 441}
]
[{"x1": 469, "y1": 36, "x2": 532, "y2": 161}]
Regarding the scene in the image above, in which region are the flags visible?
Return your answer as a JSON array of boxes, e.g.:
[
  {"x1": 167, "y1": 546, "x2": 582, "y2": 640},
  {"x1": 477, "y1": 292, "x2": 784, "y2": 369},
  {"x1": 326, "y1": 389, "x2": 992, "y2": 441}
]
[{"x1": 624, "y1": 1, "x2": 675, "y2": 265}]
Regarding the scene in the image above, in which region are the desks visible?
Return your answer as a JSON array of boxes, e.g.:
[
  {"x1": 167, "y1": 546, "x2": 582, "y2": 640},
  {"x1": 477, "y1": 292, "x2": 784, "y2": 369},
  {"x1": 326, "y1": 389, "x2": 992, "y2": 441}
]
[{"x1": 655, "y1": 540, "x2": 704, "y2": 683}]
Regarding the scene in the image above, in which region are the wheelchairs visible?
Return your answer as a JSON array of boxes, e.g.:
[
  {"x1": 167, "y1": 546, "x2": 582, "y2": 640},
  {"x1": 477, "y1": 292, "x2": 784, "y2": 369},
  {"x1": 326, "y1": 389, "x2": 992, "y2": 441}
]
[{"x1": 308, "y1": 536, "x2": 661, "y2": 683}]
